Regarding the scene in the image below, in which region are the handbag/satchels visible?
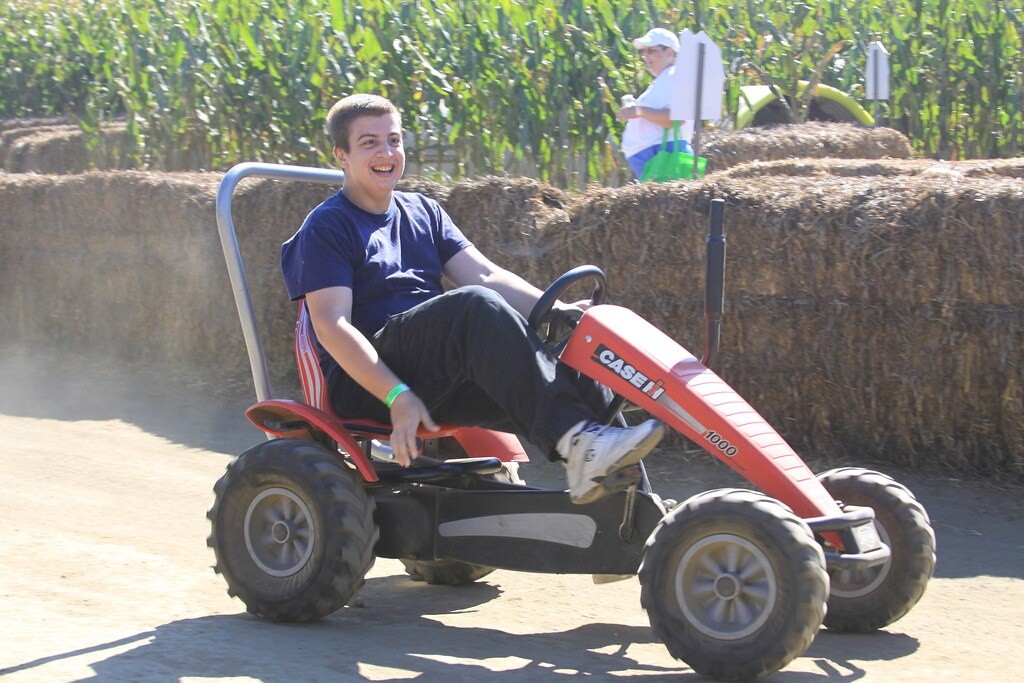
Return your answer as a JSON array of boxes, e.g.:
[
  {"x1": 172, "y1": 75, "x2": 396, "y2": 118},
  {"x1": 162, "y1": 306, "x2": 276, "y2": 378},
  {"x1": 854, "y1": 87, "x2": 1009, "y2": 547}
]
[{"x1": 641, "y1": 119, "x2": 707, "y2": 181}]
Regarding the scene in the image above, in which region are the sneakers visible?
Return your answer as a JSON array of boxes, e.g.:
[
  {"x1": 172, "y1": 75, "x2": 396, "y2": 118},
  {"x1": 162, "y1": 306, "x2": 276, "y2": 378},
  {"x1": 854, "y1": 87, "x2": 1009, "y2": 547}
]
[{"x1": 559, "y1": 418, "x2": 665, "y2": 504}]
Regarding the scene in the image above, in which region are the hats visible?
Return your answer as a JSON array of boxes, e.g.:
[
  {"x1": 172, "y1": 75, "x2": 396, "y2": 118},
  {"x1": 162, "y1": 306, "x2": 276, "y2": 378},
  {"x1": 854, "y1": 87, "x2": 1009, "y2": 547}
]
[{"x1": 631, "y1": 27, "x2": 680, "y2": 53}]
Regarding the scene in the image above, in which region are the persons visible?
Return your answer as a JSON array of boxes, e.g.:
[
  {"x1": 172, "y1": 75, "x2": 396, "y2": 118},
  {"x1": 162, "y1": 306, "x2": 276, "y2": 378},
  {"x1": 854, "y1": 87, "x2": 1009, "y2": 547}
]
[
  {"x1": 281, "y1": 93, "x2": 665, "y2": 586},
  {"x1": 617, "y1": 28, "x2": 693, "y2": 183}
]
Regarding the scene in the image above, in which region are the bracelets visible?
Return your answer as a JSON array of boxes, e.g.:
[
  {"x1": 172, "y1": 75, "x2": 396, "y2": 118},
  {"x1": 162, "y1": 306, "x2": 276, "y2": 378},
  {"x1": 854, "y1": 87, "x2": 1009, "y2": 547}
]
[
  {"x1": 636, "y1": 106, "x2": 640, "y2": 117},
  {"x1": 385, "y1": 383, "x2": 410, "y2": 407}
]
